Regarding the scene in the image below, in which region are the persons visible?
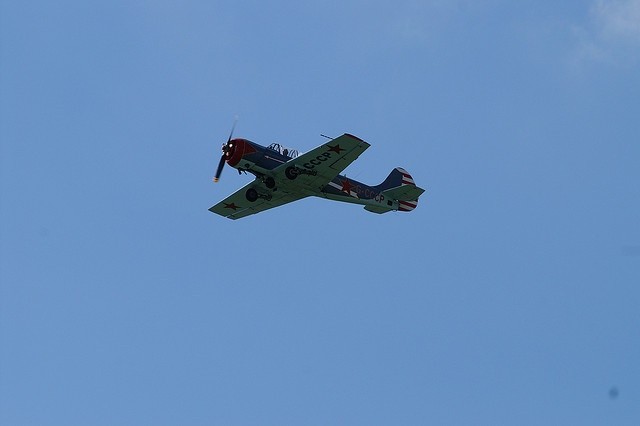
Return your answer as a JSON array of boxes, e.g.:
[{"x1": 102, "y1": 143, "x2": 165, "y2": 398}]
[{"x1": 276, "y1": 145, "x2": 291, "y2": 157}]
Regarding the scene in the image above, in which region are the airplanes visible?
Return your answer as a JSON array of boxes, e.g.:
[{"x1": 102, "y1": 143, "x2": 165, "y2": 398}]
[{"x1": 208, "y1": 115, "x2": 426, "y2": 221}]
